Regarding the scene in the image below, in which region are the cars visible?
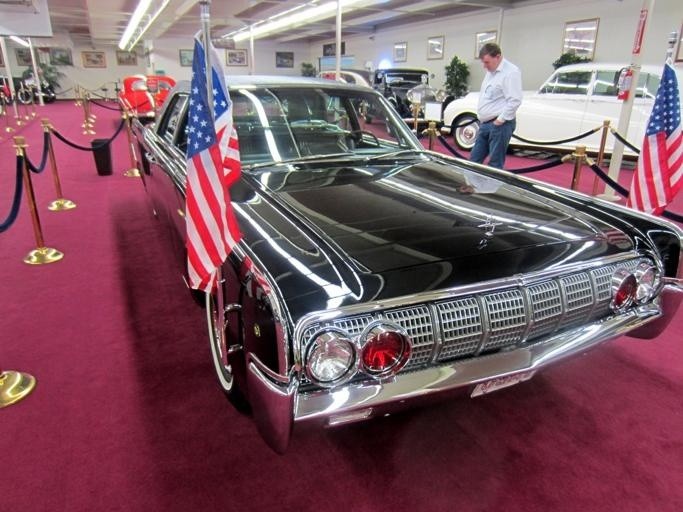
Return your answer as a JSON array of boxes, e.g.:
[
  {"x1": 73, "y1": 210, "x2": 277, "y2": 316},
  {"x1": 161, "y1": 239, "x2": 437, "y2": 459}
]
[
  {"x1": 0, "y1": 76, "x2": 56, "y2": 105},
  {"x1": 118, "y1": 74, "x2": 176, "y2": 118},
  {"x1": 130, "y1": 75, "x2": 683, "y2": 455},
  {"x1": 441, "y1": 62, "x2": 682, "y2": 160},
  {"x1": 315, "y1": 70, "x2": 372, "y2": 115}
]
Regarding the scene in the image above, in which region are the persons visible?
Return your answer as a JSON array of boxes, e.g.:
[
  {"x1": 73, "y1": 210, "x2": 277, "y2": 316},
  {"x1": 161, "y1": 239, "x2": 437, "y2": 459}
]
[
  {"x1": 469, "y1": 43, "x2": 523, "y2": 169},
  {"x1": 455, "y1": 171, "x2": 504, "y2": 196}
]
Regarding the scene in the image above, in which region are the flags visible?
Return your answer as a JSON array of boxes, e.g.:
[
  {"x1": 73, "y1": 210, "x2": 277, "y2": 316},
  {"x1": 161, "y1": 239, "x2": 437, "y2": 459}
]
[
  {"x1": 185, "y1": 31, "x2": 242, "y2": 297},
  {"x1": 625, "y1": 60, "x2": 683, "y2": 217}
]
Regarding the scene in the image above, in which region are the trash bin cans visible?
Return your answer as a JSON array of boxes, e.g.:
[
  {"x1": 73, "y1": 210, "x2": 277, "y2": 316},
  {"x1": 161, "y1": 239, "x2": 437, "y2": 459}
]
[{"x1": 92, "y1": 139, "x2": 113, "y2": 175}]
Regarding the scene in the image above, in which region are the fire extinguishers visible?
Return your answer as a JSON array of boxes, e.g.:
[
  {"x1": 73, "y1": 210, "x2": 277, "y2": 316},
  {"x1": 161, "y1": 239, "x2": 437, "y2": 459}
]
[{"x1": 614, "y1": 66, "x2": 633, "y2": 100}]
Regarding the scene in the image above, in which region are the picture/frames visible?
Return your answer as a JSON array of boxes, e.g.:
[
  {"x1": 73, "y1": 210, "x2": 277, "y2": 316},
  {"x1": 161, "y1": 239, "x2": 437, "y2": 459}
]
[
  {"x1": 561, "y1": 16, "x2": 600, "y2": 63},
  {"x1": 391, "y1": 28, "x2": 501, "y2": 63},
  {"x1": 271, "y1": 40, "x2": 347, "y2": 69},
  {"x1": 13, "y1": 46, "x2": 139, "y2": 68},
  {"x1": 178, "y1": 48, "x2": 250, "y2": 68}
]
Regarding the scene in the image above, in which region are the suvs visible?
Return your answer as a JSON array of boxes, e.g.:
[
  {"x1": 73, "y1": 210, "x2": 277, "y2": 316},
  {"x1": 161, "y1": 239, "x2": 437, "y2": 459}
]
[{"x1": 363, "y1": 68, "x2": 428, "y2": 140}]
